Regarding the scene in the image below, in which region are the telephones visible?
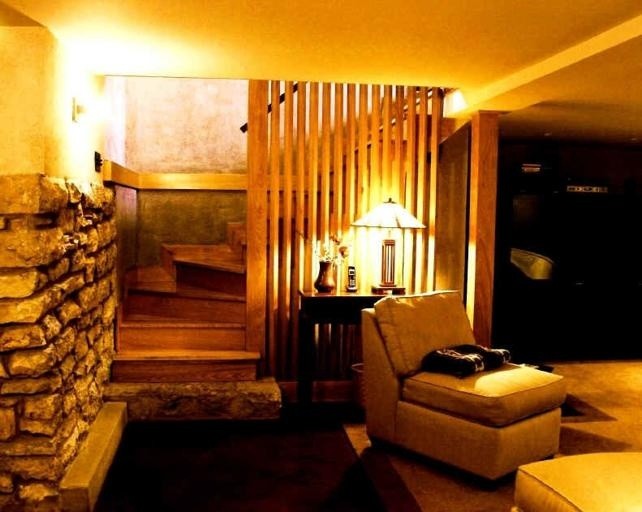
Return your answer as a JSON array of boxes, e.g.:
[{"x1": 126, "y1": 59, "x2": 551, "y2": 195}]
[{"x1": 346, "y1": 266, "x2": 357, "y2": 292}]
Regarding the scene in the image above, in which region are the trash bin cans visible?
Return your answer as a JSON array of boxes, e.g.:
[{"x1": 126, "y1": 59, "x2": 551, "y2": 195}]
[{"x1": 352, "y1": 363, "x2": 365, "y2": 407}]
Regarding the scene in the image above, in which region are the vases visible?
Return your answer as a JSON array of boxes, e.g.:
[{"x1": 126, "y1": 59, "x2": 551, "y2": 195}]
[{"x1": 314, "y1": 260, "x2": 336, "y2": 293}]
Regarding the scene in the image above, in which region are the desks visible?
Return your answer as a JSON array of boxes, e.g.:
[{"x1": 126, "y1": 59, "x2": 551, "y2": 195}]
[{"x1": 292, "y1": 290, "x2": 399, "y2": 446}]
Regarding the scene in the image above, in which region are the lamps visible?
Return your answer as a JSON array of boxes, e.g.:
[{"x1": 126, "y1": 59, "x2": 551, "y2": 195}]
[{"x1": 349, "y1": 197, "x2": 427, "y2": 296}]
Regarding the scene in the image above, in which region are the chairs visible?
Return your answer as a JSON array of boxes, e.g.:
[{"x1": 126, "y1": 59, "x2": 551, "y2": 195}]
[{"x1": 359, "y1": 289, "x2": 569, "y2": 491}]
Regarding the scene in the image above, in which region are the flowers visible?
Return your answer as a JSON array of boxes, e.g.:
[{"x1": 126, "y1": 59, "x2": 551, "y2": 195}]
[{"x1": 292, "y1": 226, "x2": 350, "y2": 263}]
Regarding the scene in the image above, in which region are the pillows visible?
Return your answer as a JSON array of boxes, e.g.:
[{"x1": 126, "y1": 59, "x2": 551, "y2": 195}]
[{"x1": 421, "y1": 338, "x2": 513, "y2": 380}]
